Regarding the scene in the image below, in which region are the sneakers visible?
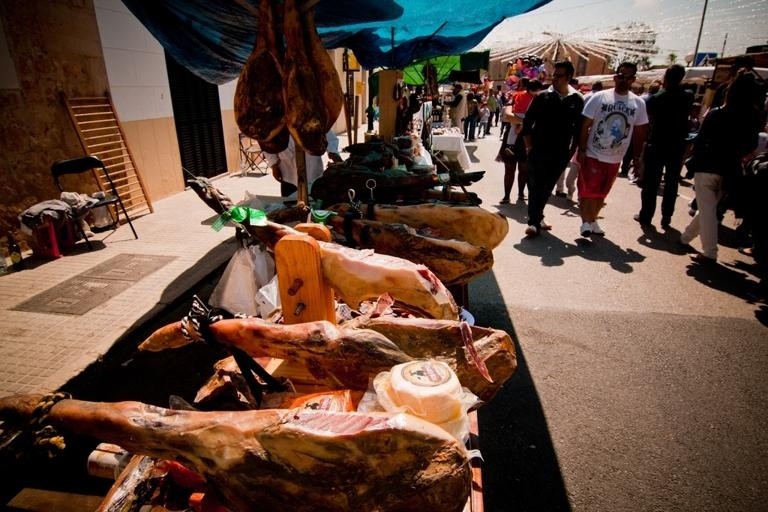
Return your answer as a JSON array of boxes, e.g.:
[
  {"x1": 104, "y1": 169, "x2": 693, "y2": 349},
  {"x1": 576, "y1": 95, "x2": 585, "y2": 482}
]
[
  {"x1": 517, "y1": 194, "x2": 525, "y2": 201},
  {"x1": 688, "y1": 208, "x2": 696, "y2": 218},
  {"x1": 524, "y1": 218, "x2": 552, "y2": 237},
  {"x1": 737, "y1": 247, "x2": 754, "y2": 257},
  {"x1": 633, "y1": 214, "x2": 642, "y2": 223},
  {"x1": 580, "y1": 220, "x2": 593, "y2": 238},
  {"x1": 589, "y1": 220, "x2": 606, "y2": 237},
  {"x1": 504, "y1": 144, "x2": 517, "y2": 157},
  {"x1": 499, "y1": 198, "x2": 511, "y2": 205}
]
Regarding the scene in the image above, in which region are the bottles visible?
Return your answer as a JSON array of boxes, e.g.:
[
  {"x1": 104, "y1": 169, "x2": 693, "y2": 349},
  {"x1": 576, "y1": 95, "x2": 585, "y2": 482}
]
[
  {"x1": 430, "y1": 107, "x2": 443, "y2": 121},
  {"x1": 8, "y1": 231, "x2": 26, "y2": 275},
  {"x1": 758, "y1": 129, "x2": 767, "y2": 151},
  {"x1": 410, "y1": 85, "x2": 452, "y2": 102},
  {"x1": 403, "y1": 120, "x2": 456, "y2": 135},
  {"x1": 0, "y1": 243, "x2": 8, "y2": 277}
]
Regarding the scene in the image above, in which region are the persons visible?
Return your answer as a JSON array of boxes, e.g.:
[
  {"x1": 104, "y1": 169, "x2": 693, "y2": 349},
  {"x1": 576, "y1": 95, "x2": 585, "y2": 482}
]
[
  {"x1": 732, "y1": 83, "x2": 768, "y2": 299},
  {"x1": 665, "y1": 72, "x2": 767, "y2": 276},
  {"x1": 575, "y1": 61, "x2": 649, "y2": 236},
  {"x1": 442, "y1": 84, "x2": 515, "y2": 143},
  {"x1": 496, "y1": 78, "x2": 544, "y2": 209},
  {"x1": 521, "y1": 60, "x2": 585, "y2": 237},
  {"x1": 688, "y1": 53, "x2": 764, "y2": 226},
  {"x1": 693, "y1": 116, "x2": 700, "y2": 131},
  {"x1": 555, "y1": 76, "x2": 663, "y2": 202},
  {"x1": 633, "y1": 63, "x2": 695, "y2": 226},
  {"x1": 511, "y1": 79, "x2": 533, "y2": 134}
]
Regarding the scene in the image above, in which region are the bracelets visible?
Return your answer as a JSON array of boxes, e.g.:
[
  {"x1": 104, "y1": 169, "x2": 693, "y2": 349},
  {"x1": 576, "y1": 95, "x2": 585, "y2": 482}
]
[{"x1": 525, "y1": 145, "x2": 532, "y2": 151}]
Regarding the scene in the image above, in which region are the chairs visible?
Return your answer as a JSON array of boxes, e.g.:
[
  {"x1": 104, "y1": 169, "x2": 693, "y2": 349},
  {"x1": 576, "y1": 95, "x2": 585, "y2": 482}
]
[{"x1": 51, "y1": 154, "x2": 138, "y2": 247}]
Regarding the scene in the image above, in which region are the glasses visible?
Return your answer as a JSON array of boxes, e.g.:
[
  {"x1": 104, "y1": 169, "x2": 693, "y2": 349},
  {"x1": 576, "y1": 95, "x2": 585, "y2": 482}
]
[
  {"x1": 551, "y1": 72, "x2": 569, "y2": 79},
  {"x1": 616, "y1": 72, "x2": 635, "y2": 82}
]
